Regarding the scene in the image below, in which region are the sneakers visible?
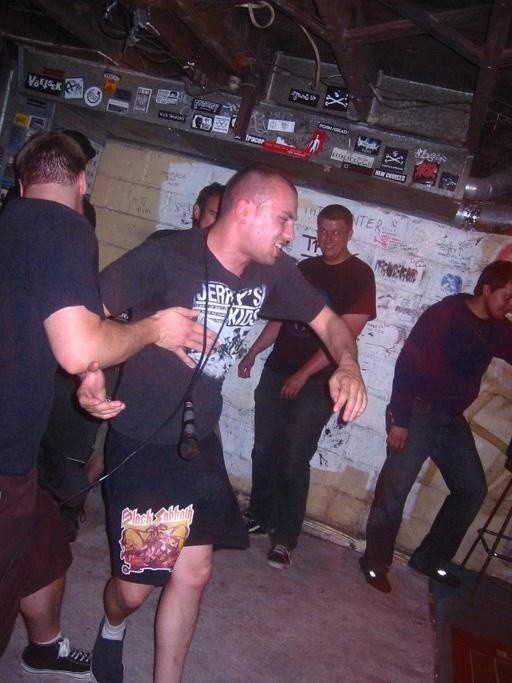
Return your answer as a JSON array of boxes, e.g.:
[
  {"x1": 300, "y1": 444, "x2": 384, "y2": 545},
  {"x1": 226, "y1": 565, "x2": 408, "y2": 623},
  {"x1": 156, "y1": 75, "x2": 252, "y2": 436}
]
[
  {"x1": 90, "y1": 616, "x2": 126, "y2": 683},
  {"x1": 242, "y1": 511, "x2": 260, "y2": 532},
  {"x1": 407, "y1": 557, "x2": 460, "y2": 589},
  {"x1": 21, "y1": 635, "x2": 92, "y2": 679},
  {"x1": 358, "y1": 557, "x2": 392, "y2": 593},
  {"x1": 265, "y1": 544, "x2": 292, "y2": 570}
]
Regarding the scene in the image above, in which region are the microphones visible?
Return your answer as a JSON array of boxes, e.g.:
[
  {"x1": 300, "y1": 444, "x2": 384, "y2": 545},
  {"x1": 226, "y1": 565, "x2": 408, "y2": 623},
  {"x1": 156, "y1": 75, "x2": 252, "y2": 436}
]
[{"x1": 178, "y1": 399, "x2": 200, "y2": 461}]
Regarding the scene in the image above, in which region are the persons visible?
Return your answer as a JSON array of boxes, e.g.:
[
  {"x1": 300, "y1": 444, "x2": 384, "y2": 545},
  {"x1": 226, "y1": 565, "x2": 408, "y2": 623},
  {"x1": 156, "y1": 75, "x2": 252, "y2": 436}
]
[
  {"x1": 1, "y1": 129, "x2": 221, "y2": 683},
  {"x1": 355, "y1": 259, "x2": 512, "y2": 593},
  {"x1": 143, "y1": 178, "x2": 229, "y2": 235},
  {"x1": 72, "y1": 162, "x2": 370, "y2": 682},
  {"x1": 236, "y1": 202, "x2": 378, "y2": 568}
]
